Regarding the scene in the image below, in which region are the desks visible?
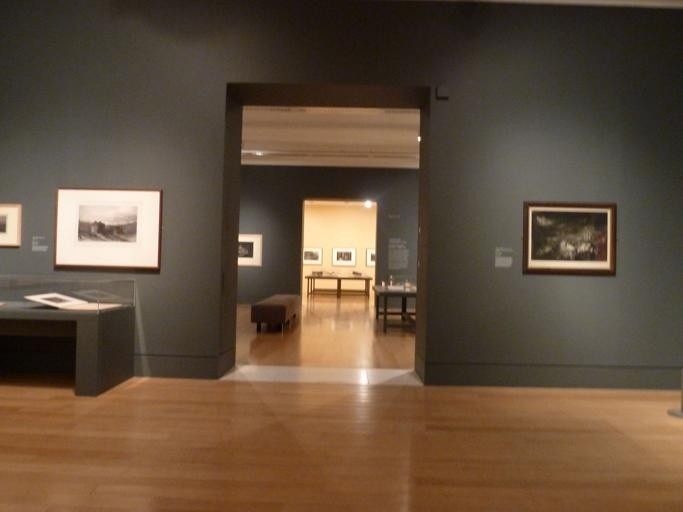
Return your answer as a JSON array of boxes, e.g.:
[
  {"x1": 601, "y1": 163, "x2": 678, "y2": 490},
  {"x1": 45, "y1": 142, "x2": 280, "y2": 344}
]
[
  {"x1": 305, "y1": 276, "x2": 373, "y2": 298},
  {"x1": 372, "y1": 285, "x2": 416, "y2": 333}
]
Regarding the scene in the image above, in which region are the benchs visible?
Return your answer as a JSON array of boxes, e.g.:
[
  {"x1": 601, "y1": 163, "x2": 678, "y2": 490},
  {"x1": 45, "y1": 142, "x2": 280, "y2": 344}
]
[
  {"x1": 0, "y1": 298, "x2": 135, "y2": 397},
  {"x1": 251, "y1": 293, "x2": 302, "y2": 335}
]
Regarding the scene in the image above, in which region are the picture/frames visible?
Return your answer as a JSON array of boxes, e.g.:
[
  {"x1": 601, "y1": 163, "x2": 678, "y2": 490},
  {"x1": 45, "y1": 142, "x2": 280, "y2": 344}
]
[
  {"x1": 523, "y1": 200, "x2": 617, "y2": 276},
  {"x1": 303, "y1": 248, "x2": 323, "y2": 265},
  {"x1": 366, "y1": 248, "x2": 376, "y2": 266},
  {"x1": 332, "y1": 248, "x2": 355, "y2": 266},
  {"x1": 0, "y1": 202, "x2": 21, "y2": 248},
  {"x1": 54, "y1": 187, "x2": 163, "y2": 271},
  {"x1": 238, "y1": 234, "x2": 263, "y2": 266}
]
